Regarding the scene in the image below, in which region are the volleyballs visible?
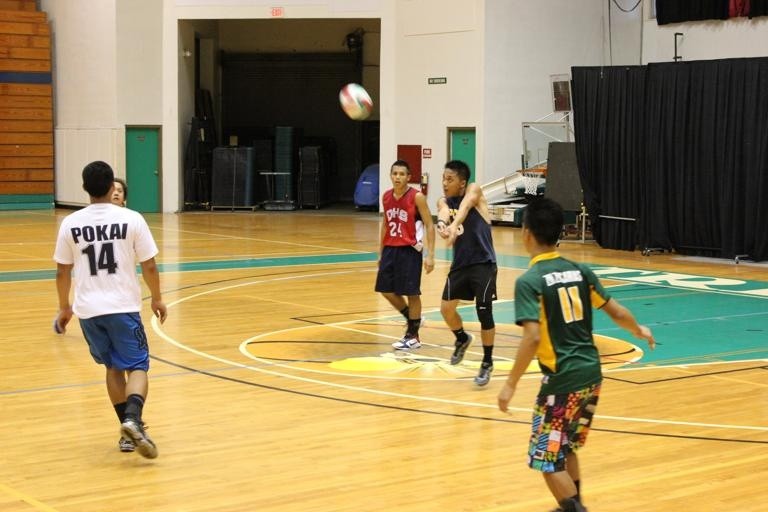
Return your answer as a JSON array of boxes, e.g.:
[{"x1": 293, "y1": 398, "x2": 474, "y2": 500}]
[{"x1": 339, "y1": 83, "x2": 373, "y2": 120}]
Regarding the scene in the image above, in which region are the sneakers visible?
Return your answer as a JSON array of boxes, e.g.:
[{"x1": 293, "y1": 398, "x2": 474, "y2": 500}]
[
  {"x1": 119, "y1": 420, "x2": 158, "y2": 458},
  {"x1": 450, "y1": 333, "x2": 472, "y2": 365},
  {"x1": 393, "y1": 334, "x2": 420, "y2": 351},
  {"x1": 474, "y1": 360, "x2": 493, "y2": 385}
]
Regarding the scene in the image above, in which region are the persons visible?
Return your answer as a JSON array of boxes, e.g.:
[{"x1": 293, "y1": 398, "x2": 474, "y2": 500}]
[
  {"x1": 435, "y1": 160, "x2": 498, "y2": 387},
  {"x1": 109, "y1": 178, "x2": 129, "y2": 208},
  {"x1": 496, "y1": 198, "x2": 656, "y2": 511},
  {"x1": 50, "y1": 159, "x2": 169, "y2": 459},
  {"x1": 373, "y1": 160, "x2": 436, "y2": 350}
]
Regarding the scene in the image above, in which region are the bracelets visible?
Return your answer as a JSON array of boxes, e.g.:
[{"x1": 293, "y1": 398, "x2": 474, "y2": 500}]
[{"x1": 434, "y1": 219, "x2": 446, "y2": 226}]
